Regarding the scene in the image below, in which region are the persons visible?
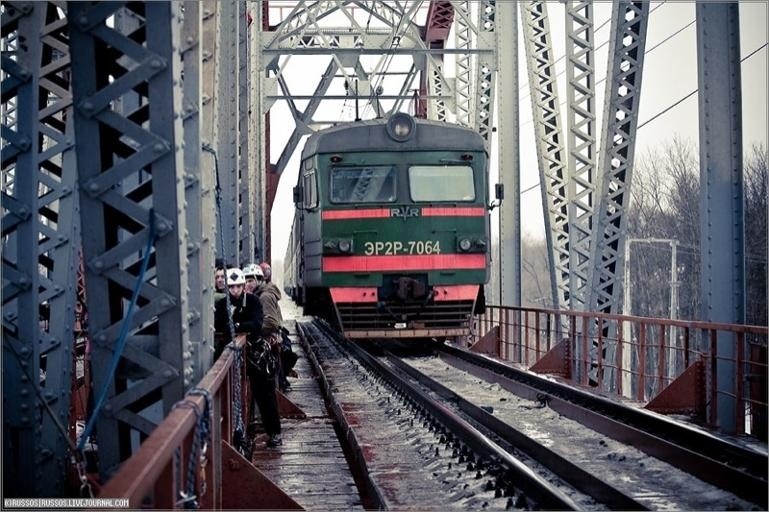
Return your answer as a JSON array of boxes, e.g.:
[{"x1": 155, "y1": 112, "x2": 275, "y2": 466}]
[{"x1": 215, "y1": 263, "x2": 294, "y2": 447}]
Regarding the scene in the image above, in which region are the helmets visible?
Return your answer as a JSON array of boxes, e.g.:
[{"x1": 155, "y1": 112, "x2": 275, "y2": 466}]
[
  {"x1": 223, "y1": 266, "x2": 246, "y2": 287},
  {"x1": 240, "y1": 263, "x2": 265, "y2": 277}
]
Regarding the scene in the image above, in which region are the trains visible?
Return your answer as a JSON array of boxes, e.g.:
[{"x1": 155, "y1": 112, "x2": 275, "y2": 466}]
[{"x1": 284, "y1": 113, "x2": 504, "y2": 341}]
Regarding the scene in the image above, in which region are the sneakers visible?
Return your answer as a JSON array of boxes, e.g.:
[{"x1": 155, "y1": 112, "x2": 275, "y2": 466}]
[{"x1": 265, "y1": 434, "x2": 283, "y2": 448}]
